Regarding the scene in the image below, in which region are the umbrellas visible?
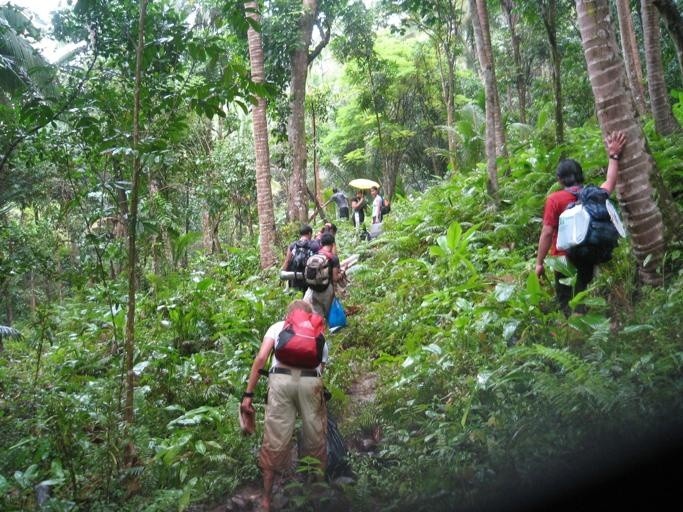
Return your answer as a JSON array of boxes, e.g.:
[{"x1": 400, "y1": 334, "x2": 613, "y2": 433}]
[{"x1": 348, "y1": 178, "x2": 380, "y2": 198}]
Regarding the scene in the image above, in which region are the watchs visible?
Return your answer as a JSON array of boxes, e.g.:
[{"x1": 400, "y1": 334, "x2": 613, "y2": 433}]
[{"x1": 610, "y1": 153, "x2": 619, "y2": 160}]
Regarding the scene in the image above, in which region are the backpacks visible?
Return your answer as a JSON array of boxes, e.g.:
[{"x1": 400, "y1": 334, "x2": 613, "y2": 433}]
[
  {"x1": 556, "y1": 186, "x2": 627, "y2": 264},
  {"x1": 291, "y1": 241, "x2": 310, "y2": 272},
  {"x1": 381, "y1": 197, "x2": 390, "y2": 214},
  {"x1": 305, "y1": 254, "x2": 333, "y2": 292},
  {"x1": 275, "y1": 309, "x2": 328, "y2": 365}
]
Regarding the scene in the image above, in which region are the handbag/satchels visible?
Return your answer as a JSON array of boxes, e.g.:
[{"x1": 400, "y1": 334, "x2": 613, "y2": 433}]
[{"x1": 368, "y1": 220, "x2": 385, "y2": 237}]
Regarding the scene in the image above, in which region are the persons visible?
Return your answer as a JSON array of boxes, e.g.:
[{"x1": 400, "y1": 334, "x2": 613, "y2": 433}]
[
  {"x1": 351, "y1": 191, "x2": 367, "y2": 226},
  {"x1": 303, "y1": 235, "x2": 348, "y2": 320},
  {"x1": 370, "y1": 186, "x2": 391, "y2": 223},
  {"x1": 281, "y1": 226, "x2": 319, "y2": 293},
  {"x1": 321, "y1": 187, "x2": 350, "y2": 220},
  {"x1": 242, "y1": 299, "x2": 330, "y2": 510},
  {"x1": 313, "y1": 223, "x2": 338, "y2": 256},
  {"x1": 536, "y1": 131, "x2": 627, "y2": 319}
]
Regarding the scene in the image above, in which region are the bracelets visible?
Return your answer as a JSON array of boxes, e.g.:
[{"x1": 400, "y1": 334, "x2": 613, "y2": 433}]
[{"x1": 243, "y1": 392, "x2": 254, "y2": 398}]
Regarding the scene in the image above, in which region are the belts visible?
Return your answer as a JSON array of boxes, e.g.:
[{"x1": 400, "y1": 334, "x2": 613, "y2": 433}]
[{"x1": 258, "y1": 367, "x2": 317, "y2": 377}]
